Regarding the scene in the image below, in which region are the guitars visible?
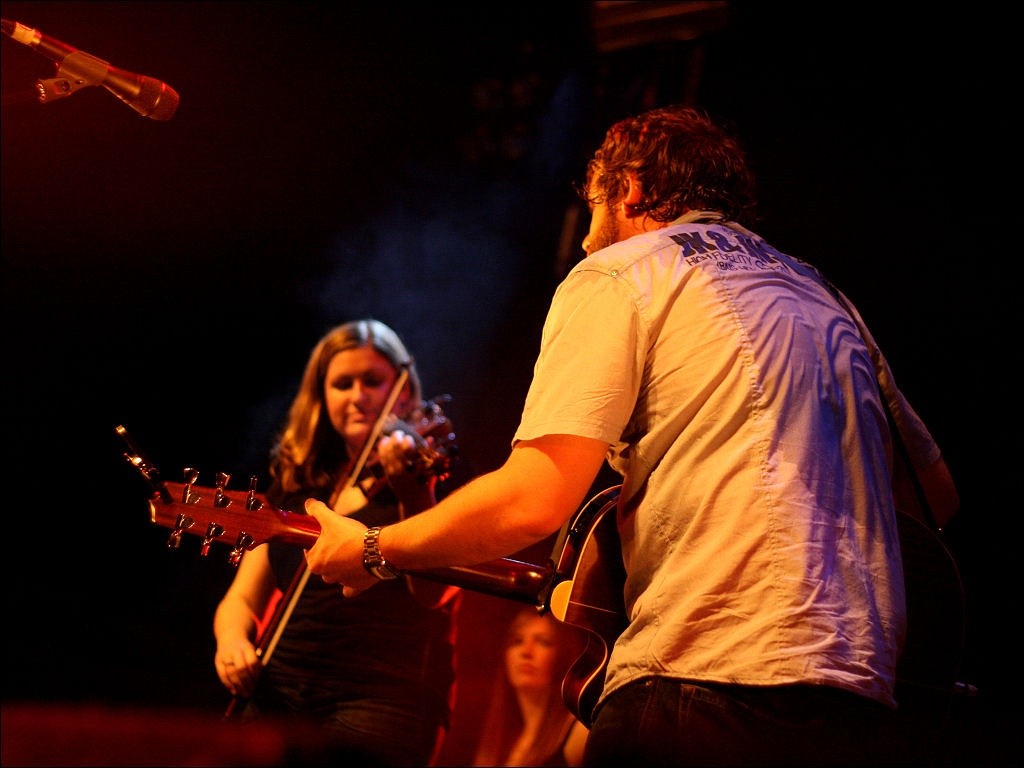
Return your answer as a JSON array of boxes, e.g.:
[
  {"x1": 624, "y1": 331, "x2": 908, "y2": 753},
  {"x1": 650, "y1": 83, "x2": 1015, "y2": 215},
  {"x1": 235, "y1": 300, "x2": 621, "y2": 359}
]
[{"x1": 143, "y1": 467, "x2": 623, "y2": 733}]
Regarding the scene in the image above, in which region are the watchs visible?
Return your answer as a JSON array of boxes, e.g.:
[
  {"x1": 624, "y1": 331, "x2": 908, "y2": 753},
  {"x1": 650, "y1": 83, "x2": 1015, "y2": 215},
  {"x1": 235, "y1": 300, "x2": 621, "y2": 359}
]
[{"x1": 362, "y1": 525, "x2": 402, "y2": 581}]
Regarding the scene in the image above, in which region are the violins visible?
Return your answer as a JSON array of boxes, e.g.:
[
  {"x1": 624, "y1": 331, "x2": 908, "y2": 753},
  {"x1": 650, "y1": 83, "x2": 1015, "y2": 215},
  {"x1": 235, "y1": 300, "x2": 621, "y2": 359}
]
[{"x1": 345, "y1": 402, "x2": 456, "y2": 485}]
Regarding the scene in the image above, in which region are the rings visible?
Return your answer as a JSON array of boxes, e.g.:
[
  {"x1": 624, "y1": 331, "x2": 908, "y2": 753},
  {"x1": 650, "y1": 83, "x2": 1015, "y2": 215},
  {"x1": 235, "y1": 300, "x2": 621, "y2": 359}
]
[{"x1": 225, "y1": 662, "x2": 234, "y2": 667}]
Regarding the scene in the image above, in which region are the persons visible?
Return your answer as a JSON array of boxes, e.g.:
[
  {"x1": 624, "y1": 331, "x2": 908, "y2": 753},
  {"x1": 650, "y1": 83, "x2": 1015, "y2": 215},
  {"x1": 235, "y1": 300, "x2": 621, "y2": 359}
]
[
  {"x1": 213, "y1": 319, "x2": 458, "y2": 768},
  {"x1": 300, "y1": 107, "x2": 956, "y2": 768},
  {"x1": 471, "y1": 606, "x2": 589, "y2": 768}
]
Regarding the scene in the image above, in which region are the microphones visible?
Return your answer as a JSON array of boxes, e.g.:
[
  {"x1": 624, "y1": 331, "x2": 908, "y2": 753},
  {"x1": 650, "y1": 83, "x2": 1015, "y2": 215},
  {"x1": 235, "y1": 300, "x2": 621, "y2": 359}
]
[{"x1": 0, "y1": 20, "x2": 181, "y2": 122}]
